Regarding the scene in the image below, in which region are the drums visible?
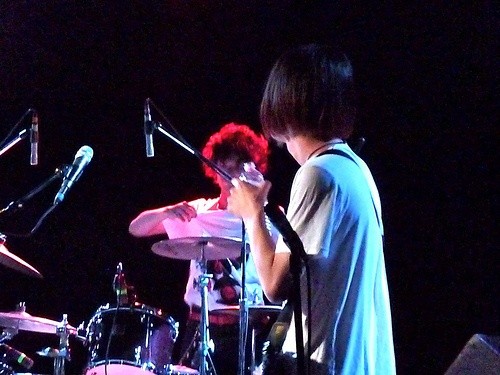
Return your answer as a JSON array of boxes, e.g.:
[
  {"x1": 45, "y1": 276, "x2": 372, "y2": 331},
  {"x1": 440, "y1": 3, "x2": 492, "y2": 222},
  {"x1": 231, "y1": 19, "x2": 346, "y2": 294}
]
[{"x1": 84, "y1": 303, "x2": 199, "y2": 375}]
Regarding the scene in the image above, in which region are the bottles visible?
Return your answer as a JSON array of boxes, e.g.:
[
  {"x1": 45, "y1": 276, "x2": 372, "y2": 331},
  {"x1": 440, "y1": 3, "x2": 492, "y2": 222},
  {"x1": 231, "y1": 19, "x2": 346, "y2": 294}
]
[{"x1": 239, "y1": 161, "x2": 269, "y2": 207}]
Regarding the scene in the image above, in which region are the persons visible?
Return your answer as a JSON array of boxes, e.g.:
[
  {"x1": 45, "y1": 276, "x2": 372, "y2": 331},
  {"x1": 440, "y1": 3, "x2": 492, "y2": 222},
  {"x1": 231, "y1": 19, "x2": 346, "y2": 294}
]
[
  {"x1": 129, "y1": 122, "x2": 284, "y2": 375},
  {"x1": 228, "y1": 46, "x2": 396, "y2": 375}
]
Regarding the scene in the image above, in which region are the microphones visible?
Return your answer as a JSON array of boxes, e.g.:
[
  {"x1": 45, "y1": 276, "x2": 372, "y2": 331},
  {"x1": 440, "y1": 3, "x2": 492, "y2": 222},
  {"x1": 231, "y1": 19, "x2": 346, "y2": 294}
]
[
  {"x1": 31, "y1": 112, "x2": 38, "y2": 165},
  {"x1": 113, "y1": 263, "x2": 127, "y2": 292},
  {"x1": 145, "y1": 100, "x2": 154, "y2": 157},
  {"x1": 54, "y1": 145, "x2": 94, "y2": 204}
]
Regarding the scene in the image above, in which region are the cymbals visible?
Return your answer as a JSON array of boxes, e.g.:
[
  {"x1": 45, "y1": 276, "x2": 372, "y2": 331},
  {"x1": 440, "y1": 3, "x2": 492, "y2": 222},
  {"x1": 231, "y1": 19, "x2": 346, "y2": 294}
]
[
  {"x1": 210, "y1": 306, "x2": 285, "y2": 319},
  {"x1": 0, "y1": 242, "x2": 41, "y2": 277},
  {"x1": 0, "y1": 312, "x2": 76, "y2": 334},
  {"x1": 150, "y1": 236, "x2": 251, "y2": 263}
]
